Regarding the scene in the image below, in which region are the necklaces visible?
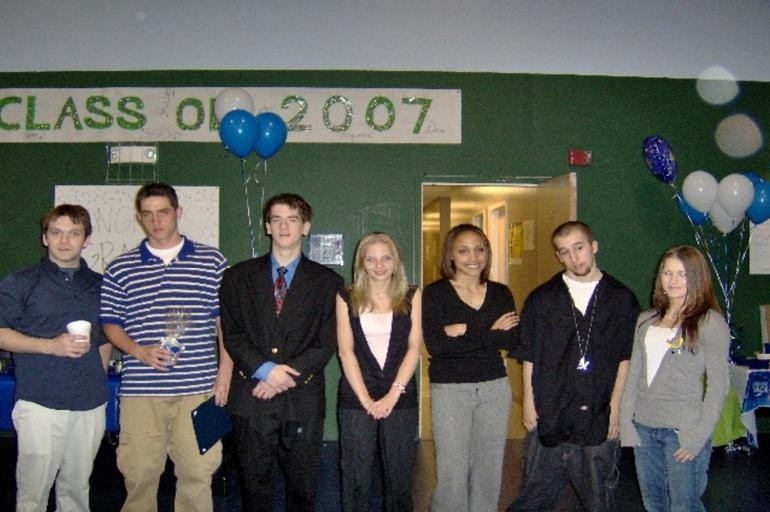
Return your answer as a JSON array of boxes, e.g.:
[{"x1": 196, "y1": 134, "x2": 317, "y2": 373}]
[{"x1": 563, "y1": 281, "x2": 600, "y2": 372}]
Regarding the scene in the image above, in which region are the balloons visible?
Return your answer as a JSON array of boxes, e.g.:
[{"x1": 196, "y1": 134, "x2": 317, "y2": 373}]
[
  {"x1": 745, "y1": 183, "x2": 770, "y2": 225},
  {"x1": 710, "y1": 201, "x2": 743, "y2": 235},
  {"x1": 213, "y1": 88, "x2": 254, "y2": 123},
  {"x1": 744, "y1": 171, "x2": 765, "y2": 183},
  {"x1": 219, "y1": 109, "x2": 254, "y2": 159},
  {"x1": 642, "y1": 133, "x2": 676, "y2": 184},
  {"x1": 255, "y1": 111, "x2": 288, "y2": 160},
  {"x1": 718, "y1": 173, "x2": 754, "y2": 216},
  {"x1": 681, "y1": 170, "x2": 718, "y2": 212},
  {"x1": 676, "y1": 192, "x2": 709, "y2": 225}
]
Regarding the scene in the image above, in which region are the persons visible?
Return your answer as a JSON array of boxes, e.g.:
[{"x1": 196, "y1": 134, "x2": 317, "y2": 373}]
[
  {"x1": 100, "y1": 181, "x2": 232, "y2": 512},
  {"x1": 0, "y1": 203, "x2": 114, "y2": 512},
  {"x1": 505, "y1": 221, "x2": 642, "y2": 511},
  {"x1": 333, "y1": 229, "x2": 423, "y2": 511},
  {"x1": 422, "y1": 223, "x2": 520, "y2": 512},
  {"x1": 217, "y1": 191, "x2": 346, "y2": 512},
  {"x1": 619, "y1": 243, "x2": 731, "y2": 512}
]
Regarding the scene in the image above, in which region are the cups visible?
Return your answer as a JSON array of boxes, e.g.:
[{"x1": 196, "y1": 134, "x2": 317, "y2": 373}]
[{"x1": 66, "y1": 319, "x2": 93, "y2": 354}]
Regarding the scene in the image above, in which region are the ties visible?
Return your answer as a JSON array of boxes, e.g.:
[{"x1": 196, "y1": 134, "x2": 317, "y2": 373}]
[{"x1": 273, "y1": 267, "x2": 288, "y2": 319}]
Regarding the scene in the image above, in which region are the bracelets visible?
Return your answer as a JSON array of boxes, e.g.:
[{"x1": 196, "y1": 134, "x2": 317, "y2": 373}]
[{"x1": 390, "y1": 381, "x2": 407, "y2": 396}]
